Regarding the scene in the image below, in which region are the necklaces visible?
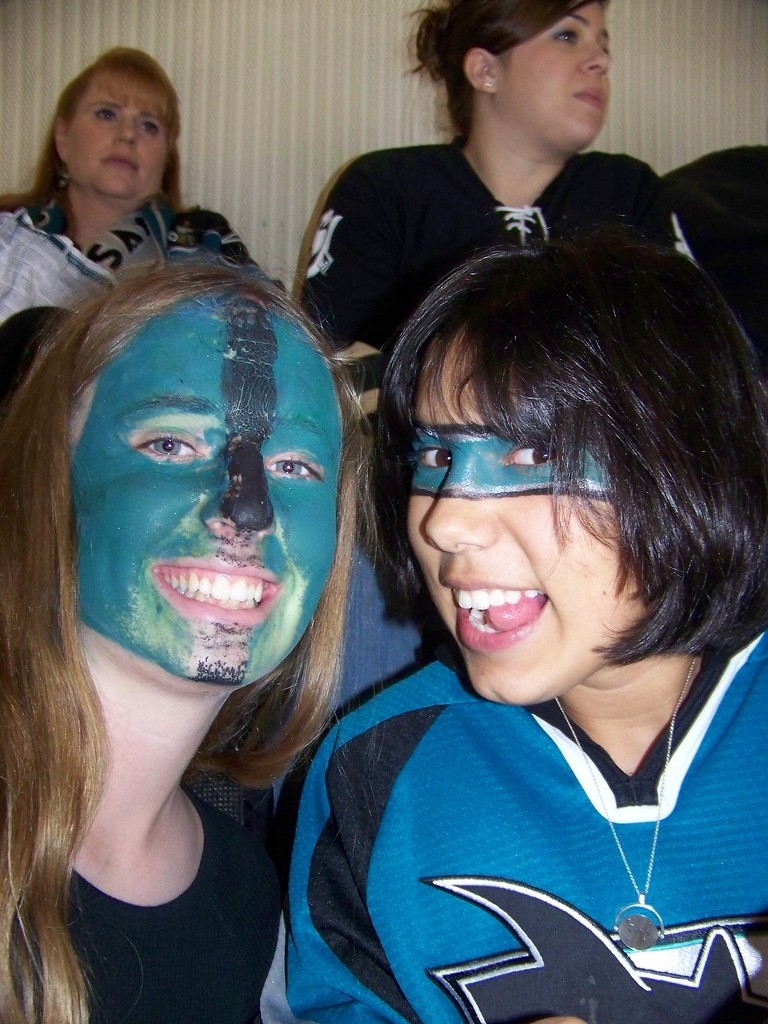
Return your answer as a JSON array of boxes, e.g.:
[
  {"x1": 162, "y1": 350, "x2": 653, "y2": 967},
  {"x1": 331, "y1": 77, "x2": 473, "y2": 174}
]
[{"x1": 555, "y1": 657, "x2": 696, "y2": 951}]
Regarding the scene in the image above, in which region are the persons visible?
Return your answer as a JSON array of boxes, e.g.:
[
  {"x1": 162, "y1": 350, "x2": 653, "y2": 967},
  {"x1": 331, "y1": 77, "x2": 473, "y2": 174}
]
[
  {"x1": 0, "y1": 49, "x2": 283, "y2": 416},
  {"x1": 300, "y1": 1, "x2": 768, "y2": 453},
  {"x1": 285, "y1": 250, "x2": 768, "y2": 1024},
  {"x1": 0, "y1": 269, "x2": 368, "y2": 1024}
]
